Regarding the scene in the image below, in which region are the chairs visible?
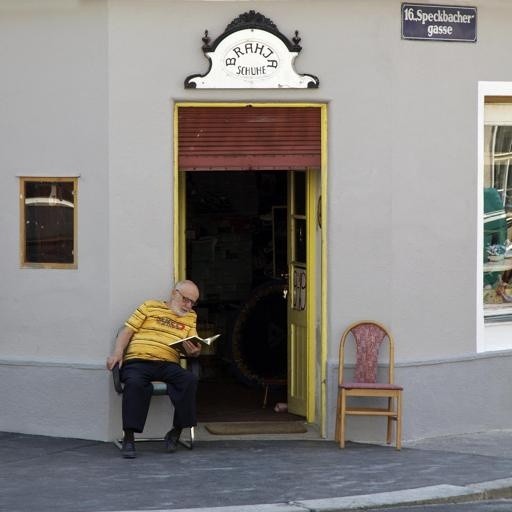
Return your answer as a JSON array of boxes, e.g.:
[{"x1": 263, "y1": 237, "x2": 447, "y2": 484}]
[
  {"x1": 335, "y1": 321, "x2": 404, "y2": 451},
  {"x1": 112, "y1": 325, "x2": 195, "y2": 452}
]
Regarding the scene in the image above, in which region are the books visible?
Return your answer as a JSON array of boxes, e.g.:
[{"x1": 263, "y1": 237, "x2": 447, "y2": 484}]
[{"x1": 167, "y1": 334, "x2": 224, "y2": 357}]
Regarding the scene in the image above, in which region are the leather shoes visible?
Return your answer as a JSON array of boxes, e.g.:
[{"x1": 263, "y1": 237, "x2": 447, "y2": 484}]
[
  {"x1": 164, "y1": 429, "x2": 180, "y2": 453},
  {"x1": 121, "y1": 440, "x2": 136, "y2": 459}
]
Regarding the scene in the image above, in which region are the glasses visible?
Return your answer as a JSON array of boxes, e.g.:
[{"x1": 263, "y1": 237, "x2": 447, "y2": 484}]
[{"x1": 174, "y1": 289, "x2": 197, "y2": 308}]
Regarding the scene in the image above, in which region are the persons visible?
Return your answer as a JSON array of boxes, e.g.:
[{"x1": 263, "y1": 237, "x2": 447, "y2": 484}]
[{"x1": 105, "y1": 279, "x2": 202, "y2": 459}]
